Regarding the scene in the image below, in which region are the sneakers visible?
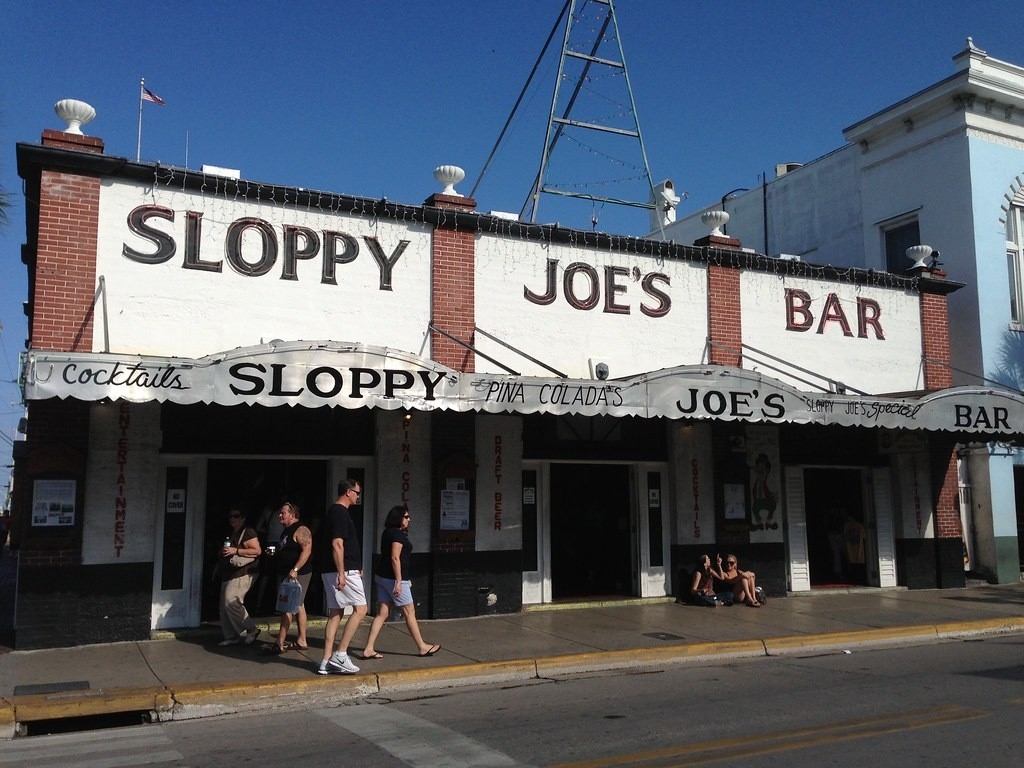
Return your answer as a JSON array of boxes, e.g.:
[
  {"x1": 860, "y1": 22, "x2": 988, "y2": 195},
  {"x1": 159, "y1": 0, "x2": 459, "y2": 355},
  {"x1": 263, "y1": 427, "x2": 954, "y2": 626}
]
[
  {"x1": 317, "y1": 661, "x2": 345, "y2": 674},
  {"x1": 329, "y1": 652, "x2": 360, "y2": 672}
]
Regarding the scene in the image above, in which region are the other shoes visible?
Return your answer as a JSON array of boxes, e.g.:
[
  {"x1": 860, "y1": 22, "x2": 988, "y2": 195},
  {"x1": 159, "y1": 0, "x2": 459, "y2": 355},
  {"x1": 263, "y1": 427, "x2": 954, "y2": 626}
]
[{"x1": 745, "y1": 599, "x2": 760, "y2": 607}]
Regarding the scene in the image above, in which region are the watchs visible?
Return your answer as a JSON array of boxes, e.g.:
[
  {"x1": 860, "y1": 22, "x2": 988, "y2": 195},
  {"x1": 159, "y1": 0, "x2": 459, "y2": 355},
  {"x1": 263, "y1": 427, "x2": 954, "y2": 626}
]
[{"x1": 292, "y1": 568, "x2": 298, "y2": 572}]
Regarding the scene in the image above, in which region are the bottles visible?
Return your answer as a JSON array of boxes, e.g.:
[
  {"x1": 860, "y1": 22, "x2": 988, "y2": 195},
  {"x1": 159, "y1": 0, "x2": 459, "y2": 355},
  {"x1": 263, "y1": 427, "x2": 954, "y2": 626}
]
[{"x1": 223, "y1": 537, "x2": 231, "y2": 559}]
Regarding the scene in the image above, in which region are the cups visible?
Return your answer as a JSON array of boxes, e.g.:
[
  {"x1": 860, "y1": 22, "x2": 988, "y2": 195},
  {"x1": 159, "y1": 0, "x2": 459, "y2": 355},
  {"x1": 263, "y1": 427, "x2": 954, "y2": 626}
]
[{"x1": 267, "y1": 547, "x2": 276, "y2": 556}]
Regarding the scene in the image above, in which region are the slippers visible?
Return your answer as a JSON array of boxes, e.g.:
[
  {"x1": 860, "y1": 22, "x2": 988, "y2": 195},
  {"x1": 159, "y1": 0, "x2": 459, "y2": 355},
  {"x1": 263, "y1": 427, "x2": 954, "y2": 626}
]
[
  {"x1": 420, "y1": 644, "x2": 441, "y2": 656},
  {"x1": 363, "y1": 653, "x2": 384, "y2": 660},
  {"x1": 261, "y1": 643, "x2": 285, "y2": 653},
  {"x1": 283, "y1": 641, "x2": 309, "y2": 650}
]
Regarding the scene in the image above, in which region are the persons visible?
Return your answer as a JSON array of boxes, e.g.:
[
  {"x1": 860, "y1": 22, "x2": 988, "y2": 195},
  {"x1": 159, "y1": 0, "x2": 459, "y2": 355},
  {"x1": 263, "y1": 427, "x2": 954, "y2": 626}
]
[
  {"x1": 823, "y1": 498, "x2": 850, "y2": 577},
  {"x1": 362, "y1": 504, "x2": 442, "y2": 658},
  {"x1": 265, "y1": 501, "x2": 314, "y2": 653},
  {"x1": 723, "y1": 554, "x2": 761, "y2": 608},
  {"x1": 691, "y1": 553, "x2": 734, "y2": 607},
  {"x1": 317, "y1": 479, "x2": 368, "y2": 675},
  {"x1": 216, "y1": 508, "x2": 262, "y2": 647}
]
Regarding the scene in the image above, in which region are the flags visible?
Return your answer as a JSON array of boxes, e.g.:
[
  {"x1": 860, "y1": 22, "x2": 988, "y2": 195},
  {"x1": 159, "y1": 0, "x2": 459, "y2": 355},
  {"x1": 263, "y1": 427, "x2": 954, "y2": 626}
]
[{"x1": 142, "y1": 86, "x2": 165, "y2": 105}]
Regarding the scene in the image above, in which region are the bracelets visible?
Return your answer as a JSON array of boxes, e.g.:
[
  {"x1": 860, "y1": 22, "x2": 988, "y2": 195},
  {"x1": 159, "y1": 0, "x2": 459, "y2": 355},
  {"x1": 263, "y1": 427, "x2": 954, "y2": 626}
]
[
  {"x1": 237, "y1": 548, "x2": 239, "y2": 556},
  {"x1": 397, "y1": 580, "x2": 403, "y2": 584}
]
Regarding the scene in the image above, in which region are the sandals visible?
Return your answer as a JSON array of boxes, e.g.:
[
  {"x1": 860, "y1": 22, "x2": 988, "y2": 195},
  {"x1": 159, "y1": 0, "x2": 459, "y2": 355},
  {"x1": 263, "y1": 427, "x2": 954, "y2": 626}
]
[
  {"x1": 244, "y1": 628, "x2": 261, "y2": 647},
  {"x1": 216, "y1": 637, "x2": 241, "y2": 646}
]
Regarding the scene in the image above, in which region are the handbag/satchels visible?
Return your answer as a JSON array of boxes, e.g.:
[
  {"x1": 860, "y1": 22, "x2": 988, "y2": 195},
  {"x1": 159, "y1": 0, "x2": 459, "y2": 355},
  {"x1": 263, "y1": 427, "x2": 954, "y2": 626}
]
[
  {"x1": 275, "y1": 577, "x2": 303, "y2": 614},
  {"x1": 213, "y1": 539, "x2": 237, "y2": 571},
  {"x1": 229, "y1": 527, "x2": 255, "y2": 567},
  {"x1": 755, "y1": 586, "x2": 767, "y2": 605},
  {"x1": 720, "y1": 598, "x2": 734, "y2": 606}
]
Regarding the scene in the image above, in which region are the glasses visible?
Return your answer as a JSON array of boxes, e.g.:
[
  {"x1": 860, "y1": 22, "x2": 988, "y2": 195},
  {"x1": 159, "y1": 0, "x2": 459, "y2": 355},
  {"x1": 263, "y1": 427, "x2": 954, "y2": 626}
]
[
  {"x1": 279, "y1": 501, "x2": 294, "y2": 511},
  {"x1": 345, "y1": 488, "x2": 360, "y2": 496},
  {"x1": 725, "y1": 560, "x2": 736, "y2": 566},
  {"x1": 402, "y1": 515, "x2": 409, "y2": 519},
  {"x1": 227, "y1": 514, "x2": 241, "y2": 519}
]
